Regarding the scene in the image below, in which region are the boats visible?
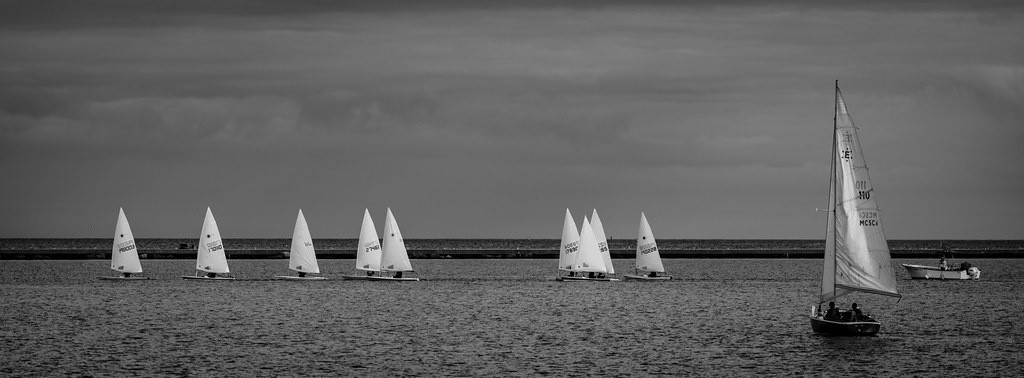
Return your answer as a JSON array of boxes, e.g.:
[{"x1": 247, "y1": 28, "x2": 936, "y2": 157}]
[{"x1": 902, "y1": 243, "x2": 982, "y2": 281}]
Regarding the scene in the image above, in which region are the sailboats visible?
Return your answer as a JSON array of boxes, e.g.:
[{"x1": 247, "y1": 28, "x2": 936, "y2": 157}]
[
  {"x1": 552, "y1": 207, "x2": 620, "y2": 283},
  {"x1": 278, "y1": 209, "x2": 329, "y2": 281},
  {"x1": 340, "y1": 207, "x2": 421, "y2": 282},
  {"x1": 181, "y1": 206, "x2": 239, "y2": 280},
  {"x1": 802, "y1": 78, "x2": 904, "y2": 335},
  {"x1": 96, "y1": 207, "x2": 158, "y2": 282},
  {"x1": 625, "y1": 211, "x2": 674, "y2": 283}
]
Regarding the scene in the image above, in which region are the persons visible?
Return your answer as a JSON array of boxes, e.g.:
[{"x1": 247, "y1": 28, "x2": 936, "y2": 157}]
[
  {"x1": 824, "y1": 302, "x2": 841, "y2": 321},
  {"x1": 939, "y1": 257, "x2": 948, "y2": 270},
  {"x1": 846, "y1": 303, "x2": 862, "y2": 321}
]
